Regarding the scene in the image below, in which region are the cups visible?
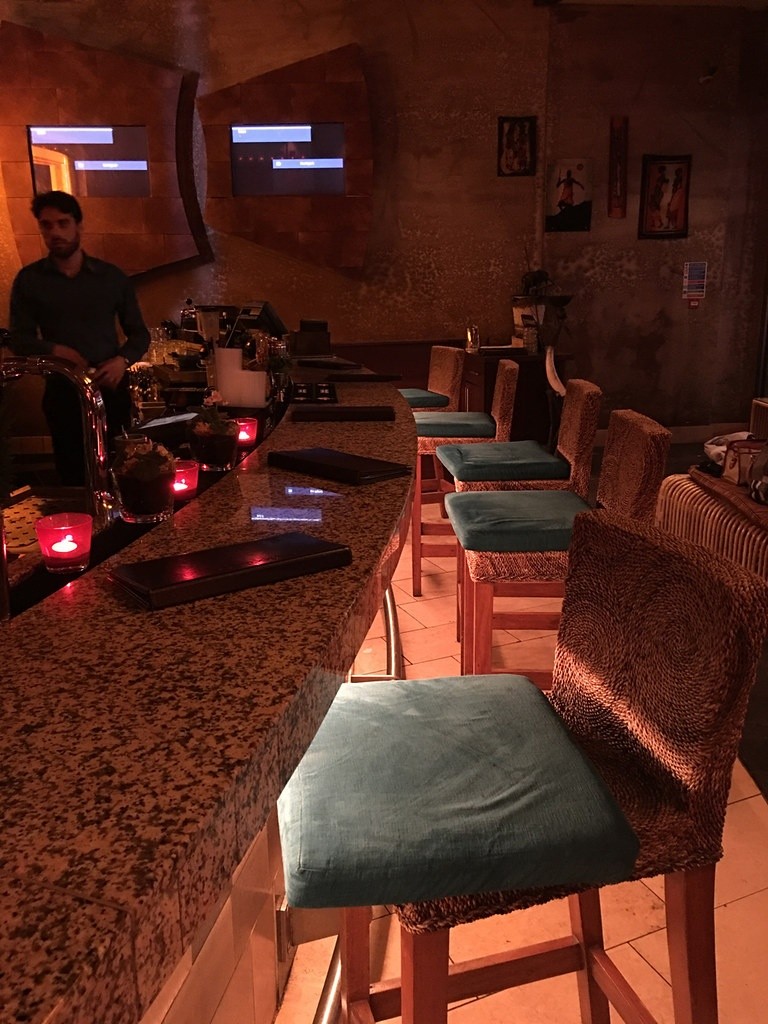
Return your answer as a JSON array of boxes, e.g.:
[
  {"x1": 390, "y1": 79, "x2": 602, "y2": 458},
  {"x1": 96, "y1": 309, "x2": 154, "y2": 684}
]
[
  {"x1": 236, "y1": 418, "x2": 257, "y2": 446},
  {"x1": 109, "y1": 467, "x2": 175, "y2": 523},
  {"x1": 114, "y1": 434, "x2": 147, "y2": 466},
  {"x1": 467, "y1": 328, "x2": 479, "y2": 347},
  {"x1": 36, "y1": 514, "x2": 93, "y2": 574},
  {"x1": 192, "y1": 431, "x2": 238, "y2": 471},
  {"x1": 175, "y1": 461, "x2": 199, "y2": 498}
]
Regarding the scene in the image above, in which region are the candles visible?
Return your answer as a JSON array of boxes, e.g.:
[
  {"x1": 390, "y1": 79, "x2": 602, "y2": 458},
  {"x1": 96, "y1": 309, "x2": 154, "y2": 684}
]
[
  {"x1": 171, "y1": 460, "x2": 201, "y2": 502},
  {"x1": 34, "y1": 513, "x2": 94, "y2": 571},
  {"x1": 235, "y1": 417, "x2": 257, "y2": 448}
]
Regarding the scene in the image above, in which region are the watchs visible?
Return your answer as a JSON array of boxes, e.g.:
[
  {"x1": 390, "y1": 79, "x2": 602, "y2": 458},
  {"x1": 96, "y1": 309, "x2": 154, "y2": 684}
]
[{"x1": 124, "y1": 355, "x2": 132, "y2": 366}]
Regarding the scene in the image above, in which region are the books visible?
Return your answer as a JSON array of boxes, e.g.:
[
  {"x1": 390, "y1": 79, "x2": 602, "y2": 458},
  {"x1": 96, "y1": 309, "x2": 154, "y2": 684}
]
[{"x1": 109, "y1": 356, "x2": 413, "y2": 611}]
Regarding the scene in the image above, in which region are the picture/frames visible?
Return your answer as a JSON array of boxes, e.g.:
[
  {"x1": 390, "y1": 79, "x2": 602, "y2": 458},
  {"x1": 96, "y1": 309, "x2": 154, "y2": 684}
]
[
  {"x1": 637, "y1": 153, "x2": 691, "y2": 240},
  {"x1": 497, "y1": 115, "x2": 537, "y2": 177}
]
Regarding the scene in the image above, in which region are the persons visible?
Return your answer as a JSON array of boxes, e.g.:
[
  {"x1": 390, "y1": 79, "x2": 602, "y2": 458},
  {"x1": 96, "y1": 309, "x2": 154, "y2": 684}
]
[
  {"x1": 556, "y1": 170, "x2": 586, "y2": 212},
  {"x1": 9, "y1": 190, "x2": 151, "y2": 463}
]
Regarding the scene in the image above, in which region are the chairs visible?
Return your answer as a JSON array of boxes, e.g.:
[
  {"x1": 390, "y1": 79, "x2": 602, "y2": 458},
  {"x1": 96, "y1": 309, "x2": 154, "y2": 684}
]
[
  {"x1": 278, "y1": 510, "x2": 768, "y2": 1024},
  {"x1": 399, "y1": 344, "x2": 464, "y2": 518},
  {"x1": 414, "y1": 357, "x2": 519, "y2": 596},
  {"x1": 436, "y1": 380, "x2": 601, "y2": 643},
  {"x1": 446, "y1": 405, "x2": 673, "y2": 691}
]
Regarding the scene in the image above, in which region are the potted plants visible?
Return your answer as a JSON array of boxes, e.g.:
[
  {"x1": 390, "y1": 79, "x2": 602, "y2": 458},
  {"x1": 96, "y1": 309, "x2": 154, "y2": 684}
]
[
  {"x1": 187, "y1": 405, "x2": 239, "y2": 472},
  {"x1": 110, "y1": 440, "x2": 175, "y2": 525}
]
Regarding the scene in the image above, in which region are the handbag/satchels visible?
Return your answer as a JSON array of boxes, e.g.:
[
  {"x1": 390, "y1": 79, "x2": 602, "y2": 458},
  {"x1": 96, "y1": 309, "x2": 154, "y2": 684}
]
[
  {"x1": 695, "y1": 431, "x2": 756, "y2": 477},
  {"x1": 721, "y1": 439, "x2": 765, "y2": 486},
  {"x1": 746, "y1": 440, "x2": 768, "y2": 504}
]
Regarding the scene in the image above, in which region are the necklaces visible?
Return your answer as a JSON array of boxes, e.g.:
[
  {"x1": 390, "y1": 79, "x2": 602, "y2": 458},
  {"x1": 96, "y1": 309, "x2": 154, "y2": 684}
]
[{"x1": 57, "y1": 264, "x2": 79, "y2": 270}]
